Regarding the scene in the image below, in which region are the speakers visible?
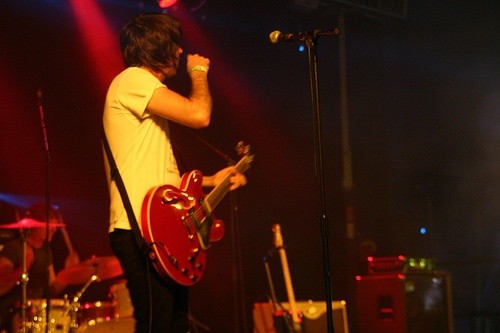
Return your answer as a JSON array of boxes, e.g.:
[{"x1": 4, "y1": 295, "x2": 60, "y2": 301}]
[{"x1": 252, "y1": 300, "x2": 349, "y2": 333}]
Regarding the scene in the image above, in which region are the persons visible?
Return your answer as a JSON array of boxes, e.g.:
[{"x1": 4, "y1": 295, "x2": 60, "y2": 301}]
[
  {"x1": 0, "y1": 198, "x2": 79, "y2": 333},
  {"x1": 101, "y1": 12, "x2": 247, "y2": 333}
]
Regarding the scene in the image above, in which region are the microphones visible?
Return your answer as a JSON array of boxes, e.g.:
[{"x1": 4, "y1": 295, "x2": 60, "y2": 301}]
[{"x1": 269, "y1": 28, "x2": 340, "y2": 44}]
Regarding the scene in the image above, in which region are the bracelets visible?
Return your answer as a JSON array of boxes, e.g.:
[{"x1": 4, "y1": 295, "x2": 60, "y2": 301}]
[{"x1": 188, "y1": 66, "x2": 207, "y2": 77}]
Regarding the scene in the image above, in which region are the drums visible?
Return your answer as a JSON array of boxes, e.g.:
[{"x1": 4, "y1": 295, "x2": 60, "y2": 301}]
[
  {"x1": 70, "y1": 303, "x2": 135, "y2": 333},
  {"x1": 14, "y1": 299, "x2": 71, "y2": 333}
]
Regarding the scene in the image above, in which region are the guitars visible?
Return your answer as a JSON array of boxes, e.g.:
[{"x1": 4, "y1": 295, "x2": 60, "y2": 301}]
[{"x1": 140, "y1": 140, "x2": 254, "y2": 286}]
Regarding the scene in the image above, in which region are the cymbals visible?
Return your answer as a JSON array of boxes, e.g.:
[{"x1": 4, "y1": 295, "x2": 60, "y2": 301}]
[
  {"x1": 0, "y1": 218, "x2": 66, "y2": 228},
  {"x1": 57, "y1": 256, "x2": 124, "y2": 284}
]
